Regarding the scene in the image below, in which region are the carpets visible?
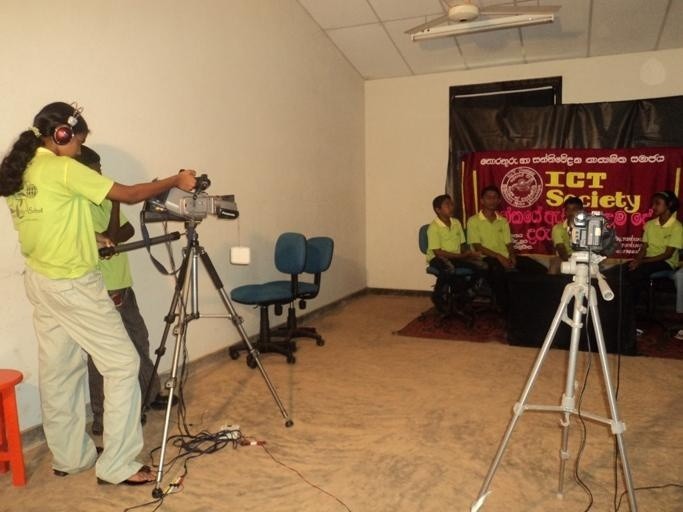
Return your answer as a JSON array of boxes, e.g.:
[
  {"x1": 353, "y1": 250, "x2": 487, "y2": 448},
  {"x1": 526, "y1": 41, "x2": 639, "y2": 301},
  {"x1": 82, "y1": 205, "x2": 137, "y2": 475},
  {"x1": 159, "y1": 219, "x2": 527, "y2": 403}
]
[{"x1": 392, "y1": 301, "x2": 682, "y2": 360}]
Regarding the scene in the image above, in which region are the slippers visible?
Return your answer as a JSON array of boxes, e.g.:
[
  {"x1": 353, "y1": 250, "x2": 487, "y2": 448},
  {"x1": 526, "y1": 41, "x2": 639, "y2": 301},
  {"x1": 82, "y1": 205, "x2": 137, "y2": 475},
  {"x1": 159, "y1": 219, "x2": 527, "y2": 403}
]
[
  {"x1": 96, "y1": 465, "x2": 158, "y2": 485},
  {"x1": 53, "y1": 446, "x2": 103, "y2": 477}
]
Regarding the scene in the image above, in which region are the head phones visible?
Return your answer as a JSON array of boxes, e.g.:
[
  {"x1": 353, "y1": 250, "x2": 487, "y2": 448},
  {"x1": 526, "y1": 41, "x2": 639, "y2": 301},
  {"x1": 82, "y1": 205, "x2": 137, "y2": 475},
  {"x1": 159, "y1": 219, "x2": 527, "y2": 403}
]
[
  {"x1": 658, "y1": 191, "x2": 680, "y2": 214},
  {"x1": 53, "y1": 101, "x2": 85, "y2": 145}
]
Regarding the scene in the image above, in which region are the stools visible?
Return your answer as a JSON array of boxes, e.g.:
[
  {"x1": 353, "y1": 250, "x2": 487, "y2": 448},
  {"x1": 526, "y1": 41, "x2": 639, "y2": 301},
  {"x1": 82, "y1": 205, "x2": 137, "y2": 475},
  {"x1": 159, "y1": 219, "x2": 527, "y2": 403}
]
[{"x1": 1, "y1": 369, "x2": 27, "y2": 487}]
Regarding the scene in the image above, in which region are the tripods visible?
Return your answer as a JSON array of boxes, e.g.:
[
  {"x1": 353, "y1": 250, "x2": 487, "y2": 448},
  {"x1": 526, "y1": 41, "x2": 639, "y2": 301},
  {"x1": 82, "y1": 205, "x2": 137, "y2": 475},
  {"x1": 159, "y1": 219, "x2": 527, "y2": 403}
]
[{"x1": 98, "y1": 230, "x2": 293, "y2": 498}]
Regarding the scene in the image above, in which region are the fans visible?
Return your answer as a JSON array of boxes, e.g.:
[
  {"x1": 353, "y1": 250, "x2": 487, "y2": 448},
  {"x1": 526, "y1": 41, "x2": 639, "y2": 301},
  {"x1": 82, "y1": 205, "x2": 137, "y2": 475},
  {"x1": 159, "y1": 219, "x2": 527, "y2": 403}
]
[{"x1": 403, "y1": 0, "x2": 562, "y2": 35}]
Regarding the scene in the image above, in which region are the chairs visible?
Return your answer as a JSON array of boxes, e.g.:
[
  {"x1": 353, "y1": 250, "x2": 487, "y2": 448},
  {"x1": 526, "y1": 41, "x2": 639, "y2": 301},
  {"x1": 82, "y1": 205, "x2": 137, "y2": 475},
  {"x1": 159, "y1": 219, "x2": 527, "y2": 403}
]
[
  {"x1": 228, "y1": 232, "x2": 334, "y2": 368},
  {"x1": 418, "y1": 224, "x2": 505, "y2": 327},
  {"x1": 637, "y1": 249, "x2": 683, "y2": 336}
]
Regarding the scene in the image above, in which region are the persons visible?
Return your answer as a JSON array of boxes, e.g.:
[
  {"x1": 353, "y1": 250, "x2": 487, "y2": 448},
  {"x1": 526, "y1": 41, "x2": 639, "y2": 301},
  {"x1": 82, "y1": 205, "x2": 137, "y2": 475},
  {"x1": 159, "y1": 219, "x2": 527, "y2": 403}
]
[
  {"x1": 68, "y1": 143, "x2": 179, "y2": 437},
  {"x1": 547, "y1": 195, "x2": 585, "y2": 275},
  {"x1": 620, "y1": 189, "x2": 682, "y2": 337},
  {"x1": 465, "y1": 185, "x2": 547, "y2": 287},
  {"x1": 1, "y1": 99, "x2": 202, "y2": 487},
  {"x1": 424, "y1": 193, "x2": 497, "y2": 319}
]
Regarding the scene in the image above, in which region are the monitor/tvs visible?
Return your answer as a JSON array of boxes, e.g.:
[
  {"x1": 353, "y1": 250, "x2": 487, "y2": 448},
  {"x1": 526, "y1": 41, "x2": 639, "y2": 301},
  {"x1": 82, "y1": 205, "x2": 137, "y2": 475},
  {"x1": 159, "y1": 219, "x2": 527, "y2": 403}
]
[{"x1": 470, "y1": 251, "x2": 637, "y2": 512}]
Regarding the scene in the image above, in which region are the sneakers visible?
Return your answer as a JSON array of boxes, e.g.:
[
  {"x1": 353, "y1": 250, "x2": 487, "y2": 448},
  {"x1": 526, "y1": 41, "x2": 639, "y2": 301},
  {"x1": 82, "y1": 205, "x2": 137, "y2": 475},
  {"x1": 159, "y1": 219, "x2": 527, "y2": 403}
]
[
  {"x1": 151, "y1": 391, "x2": 180, "y2": 411},
  {"x1": 91, "y1": 413, "x2": 148, "y2": 434}
]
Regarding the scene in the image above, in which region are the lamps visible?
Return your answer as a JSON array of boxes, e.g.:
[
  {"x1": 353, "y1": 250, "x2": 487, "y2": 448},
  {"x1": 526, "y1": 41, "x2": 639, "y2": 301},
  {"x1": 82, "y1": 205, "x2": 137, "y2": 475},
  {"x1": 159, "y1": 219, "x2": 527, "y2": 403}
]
[{"x1": 409, "y1": 14, "x2": 554, "y2": 42}]
